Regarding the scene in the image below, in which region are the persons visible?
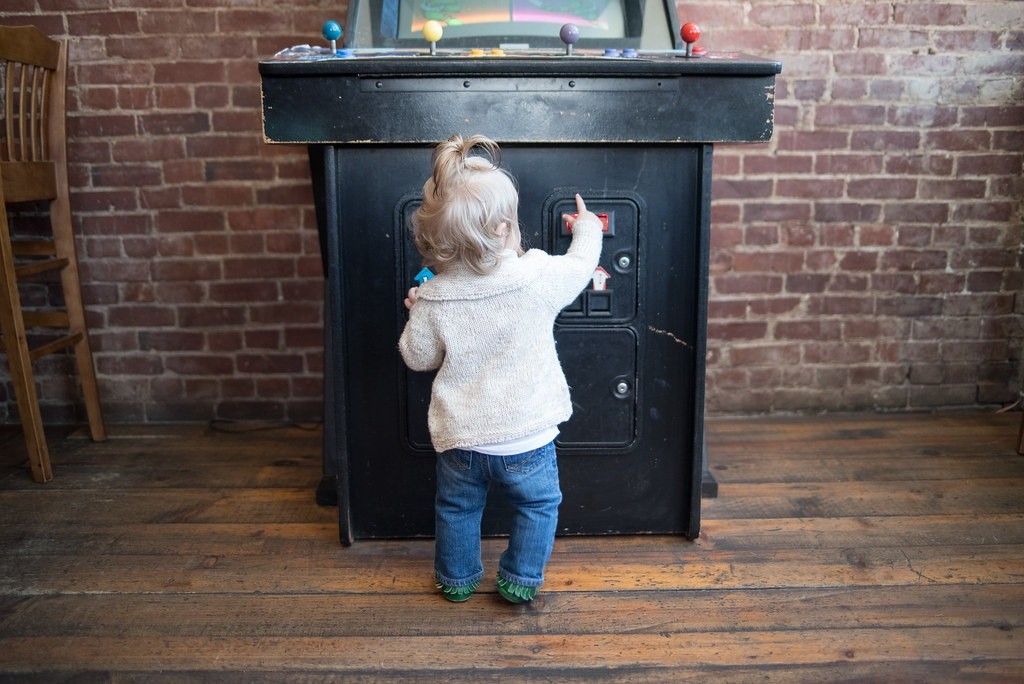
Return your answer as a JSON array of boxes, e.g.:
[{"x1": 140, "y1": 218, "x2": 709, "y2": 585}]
[{"x1": 396, "y1": 131, "x2": 604, "y2": 605}]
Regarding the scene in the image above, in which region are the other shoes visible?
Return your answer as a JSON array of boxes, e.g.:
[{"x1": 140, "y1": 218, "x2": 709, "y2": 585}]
[
  {"x1": 434, "y1": 579, "x2": 482, "y2": 602},
  {"x1": 495, "y1": 577, "x2": 537, "y2": 604}
]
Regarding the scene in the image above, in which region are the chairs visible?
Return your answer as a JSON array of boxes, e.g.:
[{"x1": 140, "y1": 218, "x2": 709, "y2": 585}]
[{"x1": 0, "y1": 24, "x2": 108, "y2": 484}]
